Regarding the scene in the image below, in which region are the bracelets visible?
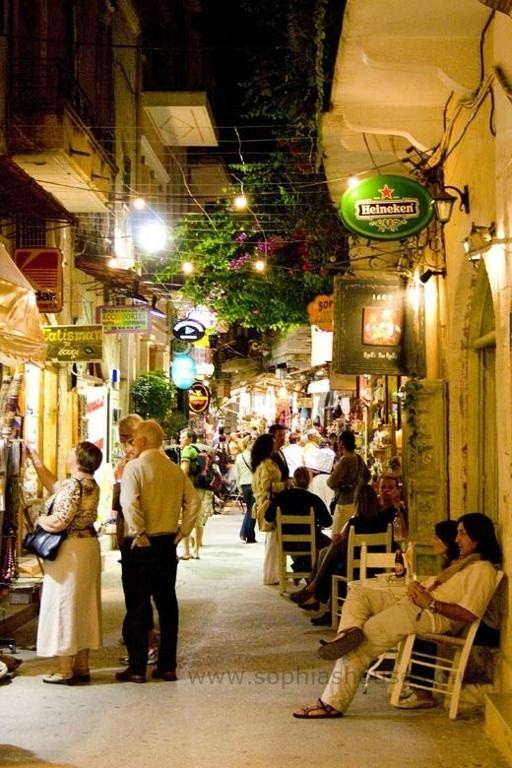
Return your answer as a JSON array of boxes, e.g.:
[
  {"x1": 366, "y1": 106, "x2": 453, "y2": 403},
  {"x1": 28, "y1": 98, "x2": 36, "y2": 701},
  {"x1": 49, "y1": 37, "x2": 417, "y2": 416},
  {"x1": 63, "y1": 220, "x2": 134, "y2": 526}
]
[
  {"x1": 31, "y1": 459, "x2": 42, "y2": 465},
  {"x1": 136, "y1": 530, "x2": 146, "y2": 539}
]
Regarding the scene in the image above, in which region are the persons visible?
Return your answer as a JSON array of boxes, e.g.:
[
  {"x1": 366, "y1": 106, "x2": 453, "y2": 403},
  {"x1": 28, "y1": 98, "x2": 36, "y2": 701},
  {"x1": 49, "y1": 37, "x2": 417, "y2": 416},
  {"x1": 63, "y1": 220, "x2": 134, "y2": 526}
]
[
  {"x1": 116, "y1": 419, "x2": 201, "y2": 683},
  {"x1": 25, "y1": 440, "x2": 105, "y2": 686},
  {"x1": 178, "y1": 418, "x2": 504, "y2": 719},
  {"x1": 113, "y1": 413, "x2": 162, "y2": 668}
]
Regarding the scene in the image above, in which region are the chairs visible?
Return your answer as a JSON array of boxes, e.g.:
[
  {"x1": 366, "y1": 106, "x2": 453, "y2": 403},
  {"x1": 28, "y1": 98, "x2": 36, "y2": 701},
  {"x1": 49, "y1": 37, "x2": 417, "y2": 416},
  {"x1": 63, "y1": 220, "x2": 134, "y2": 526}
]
[
  {"x1": 386, "y1": 572, "x2": 506, "y2": 721},
  {"x1": 331, "y1": 524, "x2": 393, "y2": 631},
  {"x1": 359, "y1": 541, "x2": 416, "y2": 579},
  {"x1": 272, "y1": 508, "x2": 317, "y2": 595}
]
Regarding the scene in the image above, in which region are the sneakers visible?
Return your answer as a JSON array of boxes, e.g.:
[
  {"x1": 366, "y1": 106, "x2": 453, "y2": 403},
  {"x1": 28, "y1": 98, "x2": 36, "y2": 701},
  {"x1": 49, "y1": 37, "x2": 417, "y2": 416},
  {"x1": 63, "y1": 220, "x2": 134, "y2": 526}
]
[{"x1": 119, "y1": 647, "x2": 161, "y2": 666}]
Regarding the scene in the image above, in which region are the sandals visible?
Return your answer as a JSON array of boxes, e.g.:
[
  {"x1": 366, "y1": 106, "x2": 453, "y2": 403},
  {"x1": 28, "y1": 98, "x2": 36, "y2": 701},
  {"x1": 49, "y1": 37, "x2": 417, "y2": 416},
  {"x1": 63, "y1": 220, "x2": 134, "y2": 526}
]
[
  {"x1": 292, "y1": 698, "x2": 344, "y2": 718},
  {"x1": 179, "y1": 555, "x2": 190, "y2": 560},
  {"x1": 190, "y1": 554, "x2": 200, "y2": 559},
  {"x1": 317, "y1": 626, "x2": 365, "y2": 661}
]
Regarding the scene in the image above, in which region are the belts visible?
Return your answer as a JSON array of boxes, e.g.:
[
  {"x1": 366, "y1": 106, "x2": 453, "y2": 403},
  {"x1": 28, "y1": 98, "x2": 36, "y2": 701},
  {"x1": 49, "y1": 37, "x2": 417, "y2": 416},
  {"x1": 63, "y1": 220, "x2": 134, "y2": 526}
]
[{"x1": 127, "y1": 531, "x2": 177, "y2": 539}]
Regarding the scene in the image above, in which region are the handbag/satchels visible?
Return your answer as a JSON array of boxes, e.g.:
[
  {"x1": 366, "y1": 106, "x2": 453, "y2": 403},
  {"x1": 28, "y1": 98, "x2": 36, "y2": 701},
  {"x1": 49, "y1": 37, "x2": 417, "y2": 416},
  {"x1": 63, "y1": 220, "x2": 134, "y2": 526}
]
[
  {"x1": 258, "y1": 497, "x2": 273, "y2": 532},
  {"x1": 337, "y1": 480, "x2": 355, "y2": 493},
  {"x1": 21, "y1": 521, "x2": 68, "y2": 561}
]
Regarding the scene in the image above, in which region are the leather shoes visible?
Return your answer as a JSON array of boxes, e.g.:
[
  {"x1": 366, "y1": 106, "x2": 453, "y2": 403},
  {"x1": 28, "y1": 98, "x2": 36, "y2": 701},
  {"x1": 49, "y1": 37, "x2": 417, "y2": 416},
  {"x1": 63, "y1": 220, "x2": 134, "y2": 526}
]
[
  {"x1": 392, "y1": 692, "x2": 436, "y2": 709},
  {"x1": 115, "y1": 670, "x2": 147, "y2": 683},
  {"x1": 152, "y1": 668, "x2": 178, "y2": 682},
  {"x1": 72, "y1": 669, "x2": 91, "y2": 683},
  {"x1": 399, "y1": 685, "x2": 417, "y2": 698},
  {"x1": 43, "y1": 671, "x2": 74, "y2": 686}
]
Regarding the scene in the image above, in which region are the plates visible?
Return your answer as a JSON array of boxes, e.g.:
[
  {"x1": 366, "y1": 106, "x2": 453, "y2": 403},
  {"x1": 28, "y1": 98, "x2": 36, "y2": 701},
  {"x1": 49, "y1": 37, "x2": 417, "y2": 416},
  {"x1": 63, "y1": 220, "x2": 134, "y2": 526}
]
[{"x1": 375, "y1": 573, "x2": 394, "y2": 578}]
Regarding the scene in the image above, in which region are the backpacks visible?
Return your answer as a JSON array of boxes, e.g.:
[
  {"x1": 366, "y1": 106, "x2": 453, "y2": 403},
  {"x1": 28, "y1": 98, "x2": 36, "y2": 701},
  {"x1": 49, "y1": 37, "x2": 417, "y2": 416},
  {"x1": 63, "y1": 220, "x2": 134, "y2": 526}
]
[{"x1": 192, "y1": 450, "x2": 224, "y2": 492}]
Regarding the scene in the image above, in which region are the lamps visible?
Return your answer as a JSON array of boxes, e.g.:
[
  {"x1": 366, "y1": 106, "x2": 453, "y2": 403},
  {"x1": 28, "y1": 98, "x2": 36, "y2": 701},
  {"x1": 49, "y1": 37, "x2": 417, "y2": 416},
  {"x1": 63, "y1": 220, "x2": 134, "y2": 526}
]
[
  {"x1": 465, "y1": 227, "x2": 512, "y2": 256},
  {"x1": 418, "y1": 269, "x2": 447, "y2": 285},
  {"x1": 435, "y1": 184, "x2": 470, "y2": 223}
]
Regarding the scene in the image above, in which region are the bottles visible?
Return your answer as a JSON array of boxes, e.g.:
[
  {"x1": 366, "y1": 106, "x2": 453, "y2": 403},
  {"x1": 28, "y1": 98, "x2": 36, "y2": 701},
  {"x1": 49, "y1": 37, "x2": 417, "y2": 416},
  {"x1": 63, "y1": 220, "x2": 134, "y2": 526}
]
[
  {"x1": 392, "y1": 514, "x2": 405, "y2": 540},
  {"x1": 395, "y1": 549, "x2": 405, "y2": 577}
]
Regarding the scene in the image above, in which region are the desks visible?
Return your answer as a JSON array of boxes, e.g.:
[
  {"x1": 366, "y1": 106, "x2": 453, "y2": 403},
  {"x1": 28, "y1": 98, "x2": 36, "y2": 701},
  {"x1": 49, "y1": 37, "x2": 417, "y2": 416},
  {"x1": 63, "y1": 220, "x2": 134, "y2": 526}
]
[{"x1": 347, "y1": 576, "x2": 433, "y2": 693}]
